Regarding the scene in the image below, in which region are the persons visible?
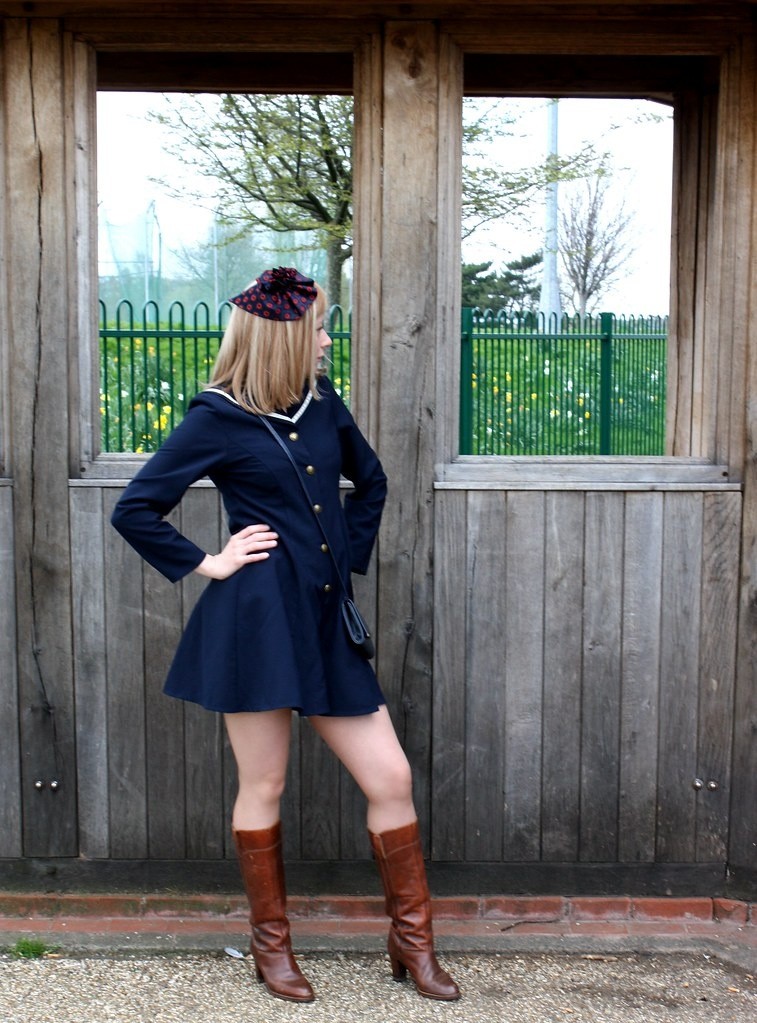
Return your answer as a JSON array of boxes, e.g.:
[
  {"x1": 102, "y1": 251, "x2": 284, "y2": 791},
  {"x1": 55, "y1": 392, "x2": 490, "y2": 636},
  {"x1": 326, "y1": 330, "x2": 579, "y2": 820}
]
[{"x1": 113, "y1": 266, "x2": 460, "y2": 1001}]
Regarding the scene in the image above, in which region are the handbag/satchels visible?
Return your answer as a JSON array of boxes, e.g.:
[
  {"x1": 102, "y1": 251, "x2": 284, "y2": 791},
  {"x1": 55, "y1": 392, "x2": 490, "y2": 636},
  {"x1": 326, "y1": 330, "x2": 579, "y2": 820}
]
[{"x1": 340, "y1": 597, "x2": 375, "y2": 661}]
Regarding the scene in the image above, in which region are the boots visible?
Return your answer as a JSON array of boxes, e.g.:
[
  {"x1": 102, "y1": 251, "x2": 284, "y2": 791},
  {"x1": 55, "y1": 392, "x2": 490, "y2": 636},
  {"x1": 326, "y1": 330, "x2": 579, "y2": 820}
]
[
  {"x1": 229, "y1": 820, "x2": 314, "y2": 1003},
  {"x1": 365, "y1": 819, "x2": 460, "y2": 1000}
]
